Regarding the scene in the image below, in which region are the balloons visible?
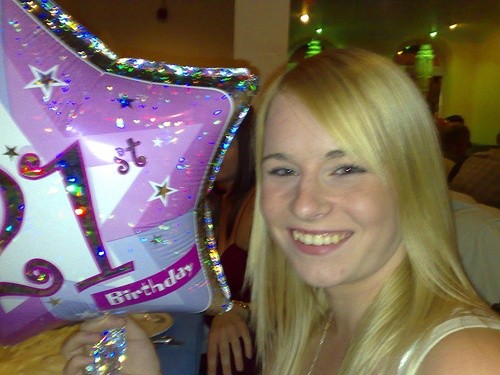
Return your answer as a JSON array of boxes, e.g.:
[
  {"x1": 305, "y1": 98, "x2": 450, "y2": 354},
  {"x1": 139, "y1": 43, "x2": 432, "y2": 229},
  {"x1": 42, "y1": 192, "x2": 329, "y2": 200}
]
[{"x1": 0, "y1": 0, "x2": 258, "y2": 345}]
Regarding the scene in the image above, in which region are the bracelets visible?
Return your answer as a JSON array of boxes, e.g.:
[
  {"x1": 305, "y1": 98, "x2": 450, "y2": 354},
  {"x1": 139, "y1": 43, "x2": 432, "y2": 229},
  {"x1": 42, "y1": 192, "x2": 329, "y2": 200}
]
[{"x1": 232, "y1": 299, "x2": 252, "y2": 324}]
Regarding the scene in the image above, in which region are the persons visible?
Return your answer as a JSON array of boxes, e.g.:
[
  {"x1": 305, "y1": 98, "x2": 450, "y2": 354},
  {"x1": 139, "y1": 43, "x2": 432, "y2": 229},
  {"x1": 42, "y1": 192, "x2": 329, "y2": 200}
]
[
  {"x1": 207, "y1": 105, "x2": 258, "y2": 374},
  {"x1": 61, "y1": 46, "x2": 500, "y2": 374},
  {"x1": 435, "y1": 114, "x2": 500, "y2": 210}
]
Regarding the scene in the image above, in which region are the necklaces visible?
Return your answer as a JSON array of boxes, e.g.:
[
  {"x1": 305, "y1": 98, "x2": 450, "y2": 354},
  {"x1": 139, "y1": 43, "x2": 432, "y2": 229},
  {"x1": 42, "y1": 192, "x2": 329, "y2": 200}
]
[{"x1": 307, "y1": 312, "x2": 333, "y2": 375}]
